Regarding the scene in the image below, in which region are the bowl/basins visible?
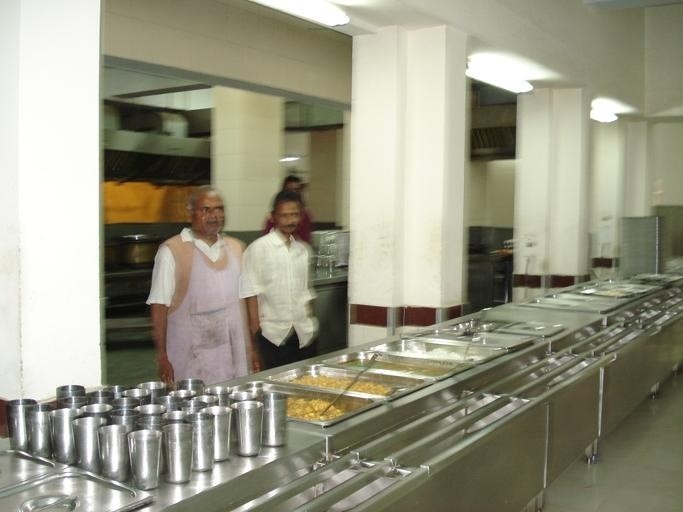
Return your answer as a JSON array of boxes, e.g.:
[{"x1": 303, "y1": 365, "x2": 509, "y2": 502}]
[
  {"x1": 262, "y1": 362, "x2": 438, "y2": 403},
  {"x1": 233, "y1": 379, "x2": 387, "y2": 426},
  {"x1": 320, "y1": 348, "x2": 473, "y2": 382},
  {"x1": 450, "y1": 317, "x2": 512, "y2": 334},
  {"x1": 370, "y1": 335, "x2": 512, "y2": 368}
]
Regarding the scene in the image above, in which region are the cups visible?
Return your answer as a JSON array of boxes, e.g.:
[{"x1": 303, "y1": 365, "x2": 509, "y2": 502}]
[
  {"x1": 25, "y1": 402, "x2": 58, "y2": 460},
  {"x1": 50, "y1": 408, "x2": 86, "y2": 464},
  {"x1": 232, "y1": 399, "x2": 264, "y2": 458},
  {"x1": 205, "y1": 386, "x2": 233, "y2": 401},
  {"x1": 235, "y1": 380, "x2": 269, "y2": 392},
  {"x1": 110, "y1": 398, "x2": 138, "y2": 408},
  {"x1": 71, "y1": 416, "x2": 108, "y2": 476},
  {"x1": 99, "y1": 423, "x2": 130, "y2": 484},
  {"x1": 178, "y1": 379, "x2": 204, "y2": 391},
  {"x1": 227, "y1": 390, "x2": 259, "y2": 443},
  {"x1": 156, "y1": 395, "x2": 181, "y2": 411},
  {"x1": 185, "y1": 411, "x2": 216, "y2": 472},
  {"x1": 137, "y1": 415, "x2": 163, "y2": 429},
  {"x1": 121, "y1": 389, "x2": 152, "y2": 404},
  {"x1": 187, "y1": 395, "x2": 220, "y2": 407},
  {"x1": 58, "y1": 395, "x2": 90, "y2": 406},
  {"x1": 126, "y1": 428, "x2": 163, "y2": 491},
  {"x1": 132, "y1": 403, "x2": 168, "y2": 417},
  {"x1": 204, "y1": 405, "x2": 232, "y2": 464},
  {"x1": 81, "y1": 403, "x2": 113, "y2": 414},
  {"x1": 55, "y1": 383, "x2": 85, "y2": 399},
  {"x1": 134, "y1": 414, "x2": 166, "y2": 429},
  {"x1": 261, "y1": 391, "x2": 290, "y2": 448},
  {"x1": 160, "y1": 422, "x2": 194, "y2": 489},
  {"x1": 162, "y1": 412, "x2": 189, "y2": 422},
  {"x1": 86, "y1": 390, "x2": 115, "y2": 404},
  {"x1": 5, "y1": 398, "x2": 40, "y2": 452},
  {"x1": 138, "y1": 381, "x2": 168, "y2": 398},
  {"x1": 107, "y1": 408, "x2": 140, "y2": 430},
  {"x1": 169, "y1": 389, "x2": 197, "y2": 399}
]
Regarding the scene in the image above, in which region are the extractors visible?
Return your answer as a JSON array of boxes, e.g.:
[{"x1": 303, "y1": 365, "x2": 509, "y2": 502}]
[{"x1": 102, "y1": 111, "x2": 210, "y2": 187}]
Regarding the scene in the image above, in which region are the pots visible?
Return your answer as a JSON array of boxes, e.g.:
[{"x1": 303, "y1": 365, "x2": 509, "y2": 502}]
[{"x1": 105, "y1": 234, "x2": 161, "y2": 265}]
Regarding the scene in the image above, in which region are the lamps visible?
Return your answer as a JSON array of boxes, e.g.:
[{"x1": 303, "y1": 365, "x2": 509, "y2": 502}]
[
  {"x1": 590, "y1": 106, "x2": 619, "y2": 125},
  {"x1": 252, "y1": 2, "x2": 350, "y2": 27},
  {"x1": 465, "y1": 63, "x2": 534, "y2": 95}
]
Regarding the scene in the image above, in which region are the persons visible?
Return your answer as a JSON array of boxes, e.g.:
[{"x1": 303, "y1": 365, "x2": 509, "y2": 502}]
[
  {"x1": 146, "y1": 185, "x2": 258, "y2": 389},
  {"x1": 241, "y1": 193, "x2": 320, "y2": 370},
  {"x1": 262, "y1": 175, "x2": 315, "y2": 244}
]
